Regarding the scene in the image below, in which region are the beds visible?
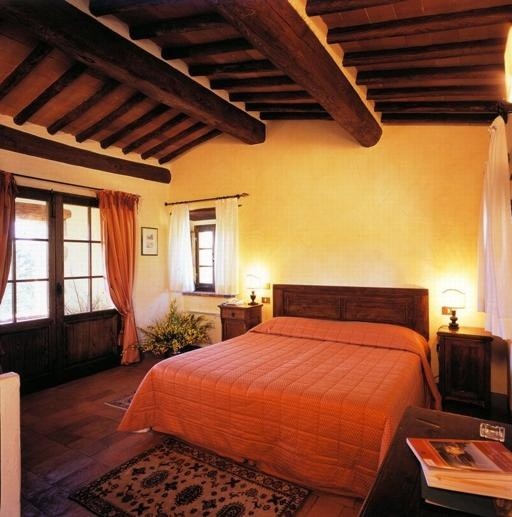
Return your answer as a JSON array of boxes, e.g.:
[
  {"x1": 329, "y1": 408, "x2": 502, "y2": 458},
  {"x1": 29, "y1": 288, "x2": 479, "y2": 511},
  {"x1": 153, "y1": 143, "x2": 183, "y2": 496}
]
[{"x1": 145, "y1": 281, "x2": 431, "y2": 500}]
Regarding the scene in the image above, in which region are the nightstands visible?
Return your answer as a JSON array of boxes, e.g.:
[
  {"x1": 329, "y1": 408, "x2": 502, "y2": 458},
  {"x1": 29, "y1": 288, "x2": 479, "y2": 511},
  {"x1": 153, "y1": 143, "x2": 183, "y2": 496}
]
[
  {"x1": 216, "y1": 301, "x2": 263, "y2": 342},
  {"x1": 436, "y1": 324, "x2": 494, "y2": 415}
]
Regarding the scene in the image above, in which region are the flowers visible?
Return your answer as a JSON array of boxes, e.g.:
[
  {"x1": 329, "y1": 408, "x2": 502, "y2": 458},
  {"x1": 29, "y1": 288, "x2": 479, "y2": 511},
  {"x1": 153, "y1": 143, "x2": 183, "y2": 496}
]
[{"x1": 122, "y1": 295, "x2": 217, "y2": 358}]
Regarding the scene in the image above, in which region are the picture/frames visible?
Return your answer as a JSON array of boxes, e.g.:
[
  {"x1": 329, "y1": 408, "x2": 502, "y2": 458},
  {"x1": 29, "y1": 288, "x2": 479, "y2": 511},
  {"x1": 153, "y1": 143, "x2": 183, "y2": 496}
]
[{"x1": 140, "y1": 226, "x2": 159, "y2": 256}]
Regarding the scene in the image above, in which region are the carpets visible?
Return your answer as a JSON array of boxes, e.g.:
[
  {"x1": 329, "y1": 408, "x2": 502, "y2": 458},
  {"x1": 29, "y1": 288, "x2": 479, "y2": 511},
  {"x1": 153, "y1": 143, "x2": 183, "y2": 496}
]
[{"x1": 66, "y1": 434, "x2": 313, "y2": 515}]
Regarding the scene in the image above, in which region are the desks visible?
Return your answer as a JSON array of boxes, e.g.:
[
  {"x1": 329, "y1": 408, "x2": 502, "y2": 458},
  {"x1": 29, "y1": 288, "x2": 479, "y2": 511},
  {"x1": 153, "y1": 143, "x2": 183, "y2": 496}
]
[{"x1": 357, "y1": 404, "x2": 511, "y2": 515}]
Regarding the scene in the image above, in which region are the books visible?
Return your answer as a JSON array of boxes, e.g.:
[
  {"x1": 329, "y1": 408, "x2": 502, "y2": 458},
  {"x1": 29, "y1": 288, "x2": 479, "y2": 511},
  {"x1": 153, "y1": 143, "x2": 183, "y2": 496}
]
[{"x1": 406, "y1": 437, "x2": 512, "y2": 500}]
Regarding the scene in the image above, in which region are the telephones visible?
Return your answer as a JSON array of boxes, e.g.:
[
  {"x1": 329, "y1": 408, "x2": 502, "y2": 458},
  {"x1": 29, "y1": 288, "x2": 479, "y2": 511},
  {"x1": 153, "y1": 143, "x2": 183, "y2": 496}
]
[{"x1": 230, "y1": 298, "x2": 243, "y2": 305}]
